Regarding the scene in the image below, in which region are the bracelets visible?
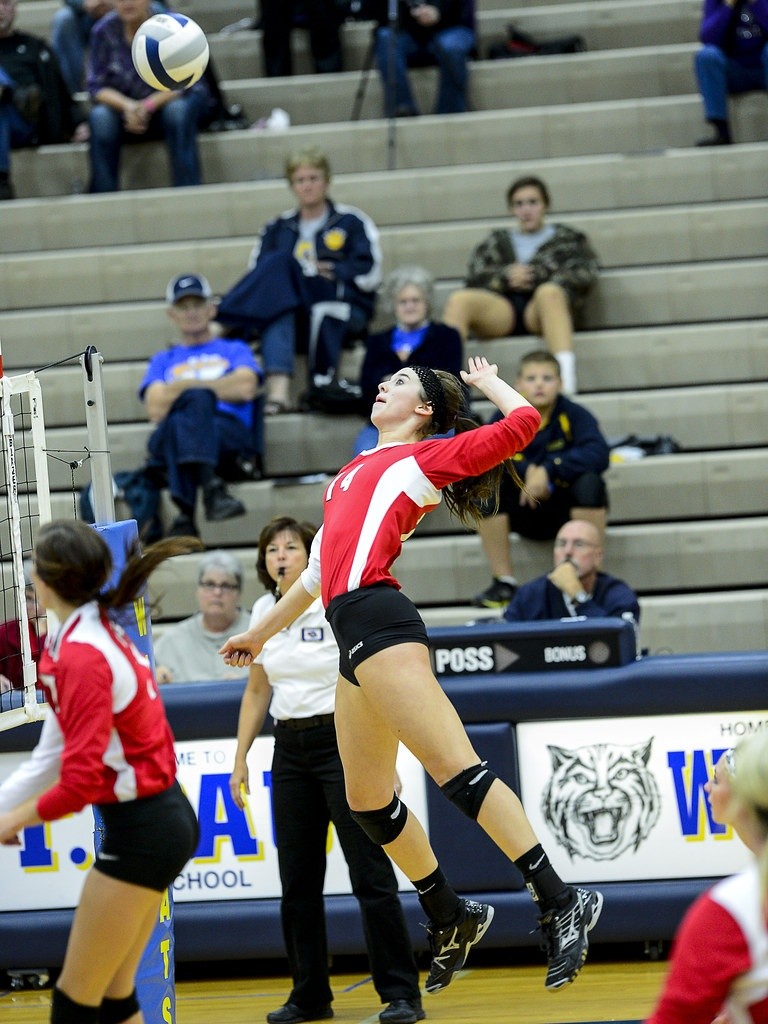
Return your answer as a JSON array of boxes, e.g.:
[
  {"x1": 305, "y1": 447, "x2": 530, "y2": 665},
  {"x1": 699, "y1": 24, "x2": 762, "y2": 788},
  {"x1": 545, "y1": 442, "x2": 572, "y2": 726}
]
[{"x1": 145, "y1": 97, "x2": 154, "y2": 113}]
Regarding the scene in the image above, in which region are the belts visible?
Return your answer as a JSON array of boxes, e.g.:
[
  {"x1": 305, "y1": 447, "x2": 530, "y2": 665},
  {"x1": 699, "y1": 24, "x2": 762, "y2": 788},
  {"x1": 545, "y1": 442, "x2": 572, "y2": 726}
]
[{"x1": 277, "y1": 713, "x2": 334, "y2": 732}]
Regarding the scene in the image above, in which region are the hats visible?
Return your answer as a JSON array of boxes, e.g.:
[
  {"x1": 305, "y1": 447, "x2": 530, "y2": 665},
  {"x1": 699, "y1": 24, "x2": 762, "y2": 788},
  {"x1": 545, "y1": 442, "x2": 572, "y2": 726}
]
[{"x1": 166, "y1": 273, "x2": 211, "y2": 304}]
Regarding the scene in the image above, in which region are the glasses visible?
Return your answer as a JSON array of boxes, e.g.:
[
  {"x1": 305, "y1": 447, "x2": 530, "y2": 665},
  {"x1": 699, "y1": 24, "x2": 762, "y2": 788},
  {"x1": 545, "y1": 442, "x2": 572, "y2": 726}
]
[{"x1": 199, "y1": 580, "x2": 240, "y2": 592}]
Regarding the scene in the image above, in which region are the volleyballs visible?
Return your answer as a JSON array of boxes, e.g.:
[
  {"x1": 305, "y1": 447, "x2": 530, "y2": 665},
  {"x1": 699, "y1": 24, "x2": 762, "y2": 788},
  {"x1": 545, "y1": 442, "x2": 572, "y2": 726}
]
[{"x1": 131, "y1": 11, "x2": 210, "y2": 92}]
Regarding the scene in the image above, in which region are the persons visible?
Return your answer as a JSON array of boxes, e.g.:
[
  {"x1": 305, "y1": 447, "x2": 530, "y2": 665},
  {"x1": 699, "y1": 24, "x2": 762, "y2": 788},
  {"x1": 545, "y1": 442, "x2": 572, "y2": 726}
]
[
  {"x1": 259, "y1": 0, "x2": 346, "y2": 79},
  {"x1": 502, "y1": 519, "x2": 641, "y2": 623},
  {"x1": 139, "y1": 272, "x2": 264, "y2": 541},
  {"x1": 229, "y1": 517, "x2": 426, "y2": 1024},
  {"x1": 0, "y1": 519, "x2": 201, "y2": 1024},
  {"x1": 0, "y1": 0, "x2": 77, "y2": 200},
  {"x1": 471, "y1": 352, "x2": 610, "y2": 608},
  {"x1": 153, "y1": 550, "x2": 251, "y2": 684},
  {"x1": 0, "y1": 559, "x2": 48, "y2": 691},
  {"x1": 246, "y1": 147, "x2": 383, "y2": 416},
  {"x1": 86, "y1": 0, "x2": 206, "y2": 194},
  {"x1": 693, "y1": 0, "x2": 768, "y2": 146},
  {"x1": 647, "y1": 724, "x2": 768, "y2": 1024},
  {"x1": 354, "y1": 265, "x2": 464, "y2": 459},
  {"x1": 442, "y1": 176, "x2": 599, "y2": 395},
  {"x1": 52, "y1": 0, "x2": 112, "y2": 89},
  {"x1": 375, "y1": 0, "x2": 479, "y2": 117},
  {"x1": 218, "y1": 356, "x2": 604, "y2": 995}
]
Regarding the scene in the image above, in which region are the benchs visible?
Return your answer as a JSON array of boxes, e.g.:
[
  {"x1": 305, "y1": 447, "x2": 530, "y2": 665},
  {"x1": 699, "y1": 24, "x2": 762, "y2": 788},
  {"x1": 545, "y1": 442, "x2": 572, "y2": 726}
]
[{"x1": 0, "y1": 0, "x2": 768, "y2": 657}]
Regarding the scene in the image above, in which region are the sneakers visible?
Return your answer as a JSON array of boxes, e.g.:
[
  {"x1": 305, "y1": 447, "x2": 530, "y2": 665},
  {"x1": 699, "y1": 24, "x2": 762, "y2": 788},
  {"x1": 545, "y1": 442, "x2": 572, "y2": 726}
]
[
  {"x1": 420, "y1": 897, "x2": 495, "y2": 994},
  {"x1": 476, "y1": 576, "x2": 519, "y2": 609},
  {"x1": 204, "y1": 485, "x2": 244, "y2": 522},
  {"x1": 267, "y1": 1002, "x2": 334, "y2": 1024},
  {"x1": 379, "y1": 998, "x2": 425, "y2": 1024},
  {"x1": 528, "y1": 887, "x2": 604, "y2": 993},
  {"x1": 169, "y1": 520, "x2": 199, "y2": 541}
]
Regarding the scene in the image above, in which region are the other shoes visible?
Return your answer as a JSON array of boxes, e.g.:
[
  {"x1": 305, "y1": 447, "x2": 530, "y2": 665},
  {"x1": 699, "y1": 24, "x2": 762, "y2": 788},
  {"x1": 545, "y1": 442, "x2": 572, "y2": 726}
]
[{"x1": 697, "y1": 133, "x2": 729, "y2": 148}]
